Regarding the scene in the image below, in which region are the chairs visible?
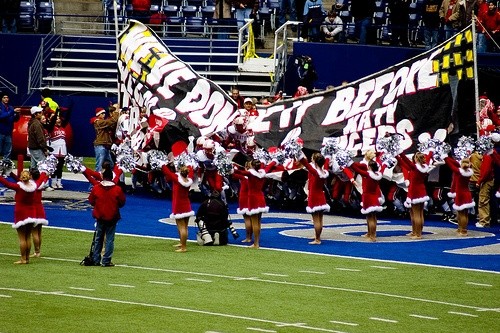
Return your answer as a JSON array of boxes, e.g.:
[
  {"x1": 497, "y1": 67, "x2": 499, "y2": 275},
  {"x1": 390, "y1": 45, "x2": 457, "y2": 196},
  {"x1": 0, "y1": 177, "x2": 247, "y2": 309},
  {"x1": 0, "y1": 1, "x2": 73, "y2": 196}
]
[
  {"x1": 108, "y1": 0, "x2": 215, "y2": 34},
  {"x1": 341, "y1": 3, "x2": 418, "y2": 44}
]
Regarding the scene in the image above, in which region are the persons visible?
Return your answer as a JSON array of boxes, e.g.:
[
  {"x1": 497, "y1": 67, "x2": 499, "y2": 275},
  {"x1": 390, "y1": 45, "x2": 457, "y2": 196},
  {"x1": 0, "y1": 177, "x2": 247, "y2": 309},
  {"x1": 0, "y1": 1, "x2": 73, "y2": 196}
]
[
  {"x1": 131, "y1": 0, "x2": 151, "y2": 23},
  {"x1": 78, "y1": 161, "x2": 125, "y2": 267},
  {"x1": 87, "y1": 103, "x2": 148, "y2": 192},
  {"x1": 0, "y1": 0, "x2": 21, "y2": 33},
  {"x1": 27, "y1": 91, "x2": 71, "y2": 192},
  {"x1": 232, "y1": 86, "x2": 310, "y2": 116},
  {"x1": 297, "y1": 152, "x2": 330, "y2": 245},
  {"x1": 444, "y1": 95, "x2": 500, "y2": 236},
  {"x1": 349, "y1": 0, "x2": 500, "y2": 53},
  {"x1": 333, "y1": 147, "x2": 435, "y2": 242},
  {"x1": 232, "y1": 0, "x2": 345, "y2": 43},
  {"x1": 0, "y1": 168, "x2": 49, "y2": 263},
  {"x1": 158, "y1": 117, "x2": 306, "y2": 252},
  {"x1": 0, "y1": 93, "x2": 21, "y2": 158}
]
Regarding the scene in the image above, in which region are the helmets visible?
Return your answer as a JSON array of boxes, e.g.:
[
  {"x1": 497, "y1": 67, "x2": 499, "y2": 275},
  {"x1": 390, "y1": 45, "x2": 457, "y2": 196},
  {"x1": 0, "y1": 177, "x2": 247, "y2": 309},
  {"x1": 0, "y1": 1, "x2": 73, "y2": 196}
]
[
  {"x1": 202, "y1": 139, "x2": 216, "y2": 152},
  {"x1": 246, "y1": 136, "x2": 256, "y2": 146},
  {"x1": 196, "y1": 136, "x2": 205, "y2": 145},
  {"x1": 234, "y1": 117, "x2": 248, "y2": 129}
]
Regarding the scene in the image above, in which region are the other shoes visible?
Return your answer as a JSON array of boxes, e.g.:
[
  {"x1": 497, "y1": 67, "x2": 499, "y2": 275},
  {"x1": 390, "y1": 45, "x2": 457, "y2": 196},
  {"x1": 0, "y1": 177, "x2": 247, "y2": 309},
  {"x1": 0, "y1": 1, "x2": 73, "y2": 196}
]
[
  {"x1": 45, "y1": 186, "x2": 55, "y2": 191},
  {"x1": 475, "y1": 222, "x2": 485, "y2": 228},
  {"x1": 101, "y1": 262, "x2": 115, "y2": 267},
  {"x1": 125, "y1": 189, "x2": 131, "y2": 194},
  {"x1": 448, "y1": 219, "x2": 459, "y2": 225},
  {"x1": 426, "y1": 209, "x2": 480, "y2": 223}
]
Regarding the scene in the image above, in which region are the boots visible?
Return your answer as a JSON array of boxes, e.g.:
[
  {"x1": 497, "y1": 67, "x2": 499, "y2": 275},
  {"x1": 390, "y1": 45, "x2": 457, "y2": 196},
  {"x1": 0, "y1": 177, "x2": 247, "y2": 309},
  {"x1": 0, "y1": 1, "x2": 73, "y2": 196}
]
[
  {"x1": 199, "y1": 219, "x2": 213, "y2": 245},
  {"x1": 57, "y1": 179, "x2": 63, "y2": 189},
  {"x1": 51, "y1": 178, "x2": 58, "y2": 189},
  {"x1": 227, "y1": 214, "x2": 239, "y2": 238}
]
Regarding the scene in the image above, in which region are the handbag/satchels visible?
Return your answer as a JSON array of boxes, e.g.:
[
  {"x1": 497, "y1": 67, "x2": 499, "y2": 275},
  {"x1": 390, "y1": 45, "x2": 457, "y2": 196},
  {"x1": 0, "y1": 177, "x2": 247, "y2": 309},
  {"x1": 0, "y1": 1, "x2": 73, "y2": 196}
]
[{"x1": 79, "y1": 255, "x2": 94, "y2": 266}]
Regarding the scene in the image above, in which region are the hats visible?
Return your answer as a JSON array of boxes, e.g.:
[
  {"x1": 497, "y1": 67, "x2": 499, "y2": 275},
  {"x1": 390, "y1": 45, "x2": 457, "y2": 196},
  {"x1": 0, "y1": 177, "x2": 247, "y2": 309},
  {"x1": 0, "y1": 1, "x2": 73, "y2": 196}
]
[
  {"x1": 244, "y1": 98, "x2": 253, "y2": 104},
  {"x1": 96, "y1": 108, "x2": 106, "y2": 116},
  {"x1": 0, "y1": 91, "x2": 10, "y2": 99},
  {"x1": 41, "y1": 88, "x2": 52, "y2": 97},
  {"x1": 30, "y1": 106, "x2": 43, "y2": 114}
]
